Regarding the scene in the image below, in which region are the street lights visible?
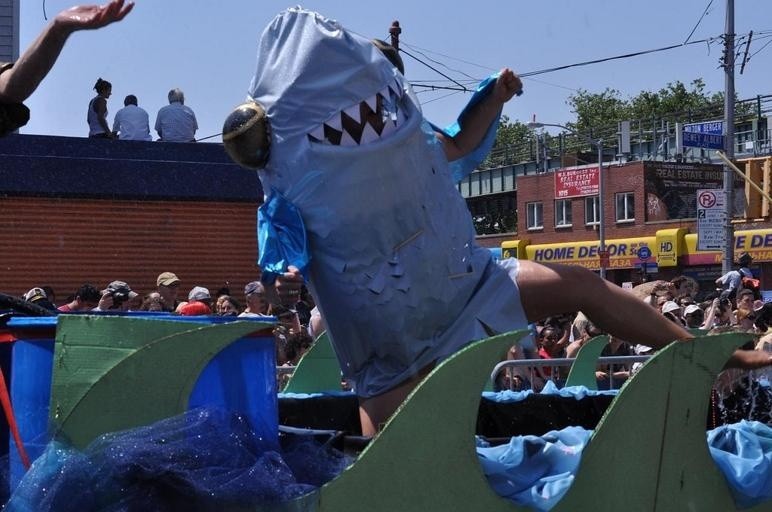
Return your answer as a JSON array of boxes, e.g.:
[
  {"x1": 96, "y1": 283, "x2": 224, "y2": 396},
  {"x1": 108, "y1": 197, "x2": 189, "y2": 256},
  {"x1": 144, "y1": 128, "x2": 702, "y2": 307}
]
[{"x1": 527, "y1": 122, "x2": 606, "y2": 278}]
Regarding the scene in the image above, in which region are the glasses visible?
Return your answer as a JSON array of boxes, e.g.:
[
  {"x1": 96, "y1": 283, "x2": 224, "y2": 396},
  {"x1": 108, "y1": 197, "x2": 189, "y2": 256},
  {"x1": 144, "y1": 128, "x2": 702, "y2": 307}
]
[
  {"x1": 680, "y1": 302, "x2": 696, "y2": 306},
  {"x1": 585, "y1": 327, "x2": 600, "y2": 338}
]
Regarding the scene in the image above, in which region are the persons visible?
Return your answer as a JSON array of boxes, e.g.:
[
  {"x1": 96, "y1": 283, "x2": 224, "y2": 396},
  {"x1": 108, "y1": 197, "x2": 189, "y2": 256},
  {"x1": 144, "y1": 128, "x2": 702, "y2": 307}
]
[
  {"x1": 221, "y1": 5, "x2": 772, "y2": 437},
  {"x1": 87, "y1": 78, "x2": 119, "y2": 142},
  {"x1": 340, "y1": 371, "x2": 354, "y2": 393},
  {"x1": 154, "y1": 88, "x2": 199, "y2": 142},
  {"x1": 0, "y1": 0, "x2": 132, "y2": 133},
  {"x1": 241, "y1": 265, "x2": 324, "y2": 390},
  {"x1": 112, "y1": 94, "x2": 152, "y2": 141},
  {"x1": 24, "y1": 272, "x2": 241, "y2": 315},
  {"x1": 497, "y1": 254, "x2": 772, "y2": 425}
]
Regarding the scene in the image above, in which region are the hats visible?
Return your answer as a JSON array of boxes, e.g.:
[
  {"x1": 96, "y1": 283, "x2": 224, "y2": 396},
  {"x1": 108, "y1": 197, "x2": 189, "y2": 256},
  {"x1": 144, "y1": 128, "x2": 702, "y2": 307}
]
[
  {"x1": 737, "y1": 252, "x2": 755, "y2": 264},
  {"x1": 659, "y1": 300, "x2": 769, "y2": 317},
  {"x1": 25, "y1": 272, "x2": 299, "y2": 318}
]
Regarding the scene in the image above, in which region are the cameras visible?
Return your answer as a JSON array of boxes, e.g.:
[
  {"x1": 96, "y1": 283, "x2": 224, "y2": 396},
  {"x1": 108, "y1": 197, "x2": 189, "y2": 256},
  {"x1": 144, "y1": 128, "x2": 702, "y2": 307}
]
[
  {"x1": 105, "y1": 288, "x2": 129, "y2": 309},
  {"x1": 660, "y1": 284, "x2": 668, "y2": 289},
  {"x1": 717, "y1": 297, "x2": 728, "y2": 312}
]
